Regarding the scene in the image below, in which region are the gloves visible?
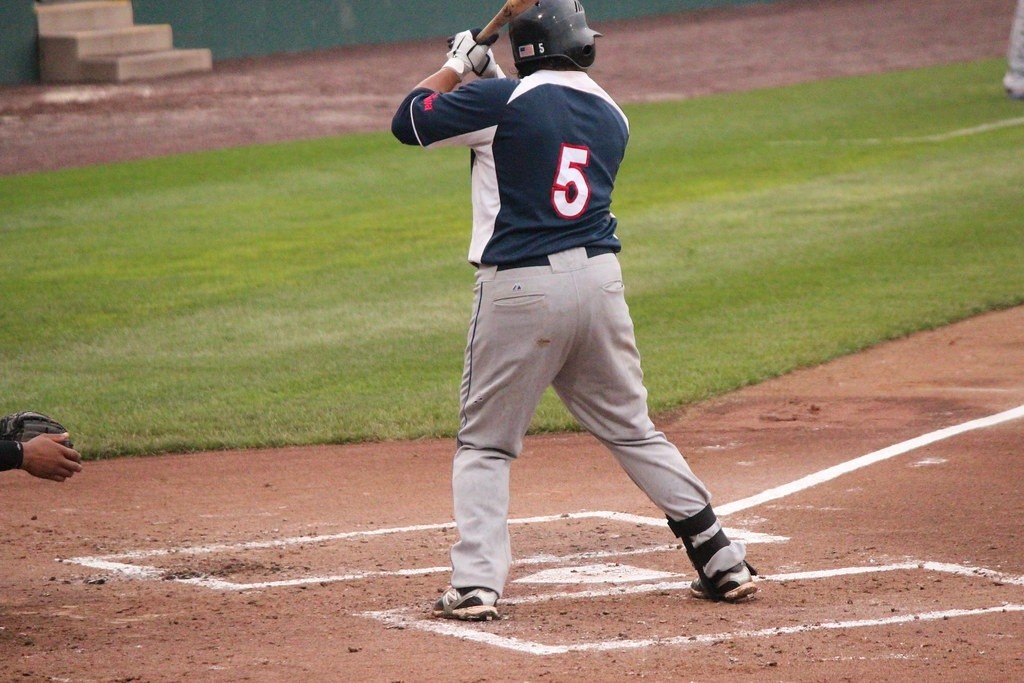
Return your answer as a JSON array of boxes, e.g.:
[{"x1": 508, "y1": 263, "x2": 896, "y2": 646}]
[{"x1": 440, "y1": 28, "x2": 506, "y2": 82}]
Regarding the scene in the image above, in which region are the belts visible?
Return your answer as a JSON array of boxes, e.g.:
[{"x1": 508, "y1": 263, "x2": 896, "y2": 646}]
[{"x1": 495, "y1": 244, "x2": 616, "y2": 272}]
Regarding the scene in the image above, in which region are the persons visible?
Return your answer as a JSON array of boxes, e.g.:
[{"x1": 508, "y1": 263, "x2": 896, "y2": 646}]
[
  {"x1": 0, "y1": 412, "x2": 84, "y2": 483},
  {"x1": 1002, "y1": 0, "x2": 1023, "y2": 97},
  {"x1": 390, "y1": 0, "x2": 758, "y2": 620}
]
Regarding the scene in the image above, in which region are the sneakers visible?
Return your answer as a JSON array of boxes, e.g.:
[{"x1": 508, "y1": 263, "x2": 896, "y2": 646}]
[
  {"x1": 691, "y1": 562, "x2": 757, "y2": 599},
  {"x1": 434, "y1": 588, "x2": 500, "y2": 620}
]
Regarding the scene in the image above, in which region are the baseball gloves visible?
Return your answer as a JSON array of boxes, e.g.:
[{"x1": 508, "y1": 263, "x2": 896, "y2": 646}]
[{"x1": 0, "y1": 409, "x2": 74, "y2": 453}]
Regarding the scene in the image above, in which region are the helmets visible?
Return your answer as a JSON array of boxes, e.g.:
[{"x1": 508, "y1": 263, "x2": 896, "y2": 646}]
[{"x1": 508, "y1": 0, "x2": 604, "y2": 69}]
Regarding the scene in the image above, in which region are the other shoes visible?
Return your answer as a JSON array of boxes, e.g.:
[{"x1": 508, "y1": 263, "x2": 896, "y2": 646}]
[{"x1": 1002, "y1": 70, "x2": 1024, "y2": 99}]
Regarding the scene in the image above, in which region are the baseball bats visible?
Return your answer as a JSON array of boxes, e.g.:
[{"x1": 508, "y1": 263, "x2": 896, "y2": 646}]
[{"x1": 473, "y1": 0, "x2": 536, "y2": 44}]
